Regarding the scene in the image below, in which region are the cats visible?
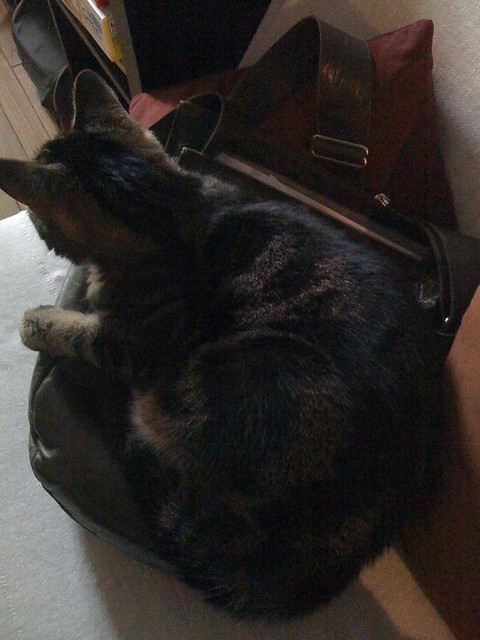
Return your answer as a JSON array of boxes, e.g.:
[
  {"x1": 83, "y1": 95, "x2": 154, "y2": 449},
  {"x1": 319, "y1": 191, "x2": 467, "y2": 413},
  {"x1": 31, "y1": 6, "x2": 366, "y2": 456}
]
[{"x1": 0, "y1": 67, "x2": 447, "y2": 624}]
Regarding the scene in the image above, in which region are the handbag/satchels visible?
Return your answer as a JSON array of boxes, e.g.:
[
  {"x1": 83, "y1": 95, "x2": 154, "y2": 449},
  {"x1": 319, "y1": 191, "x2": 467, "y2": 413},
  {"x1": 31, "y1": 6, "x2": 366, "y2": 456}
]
[{"x1": 29, "y1": 15, "x2": 478, "y2": 580}]
